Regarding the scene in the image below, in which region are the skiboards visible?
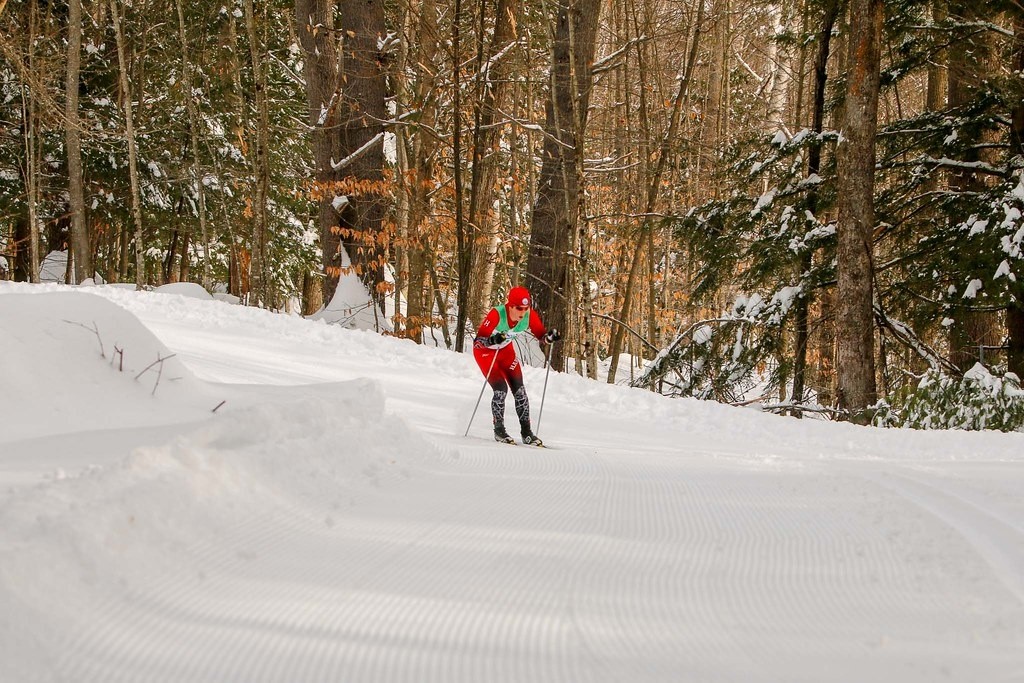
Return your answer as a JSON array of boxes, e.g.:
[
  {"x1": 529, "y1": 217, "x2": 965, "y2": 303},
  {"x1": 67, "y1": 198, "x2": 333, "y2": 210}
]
[{"x1": 468, "y1": 435, "x2": 565, "y2": 451}]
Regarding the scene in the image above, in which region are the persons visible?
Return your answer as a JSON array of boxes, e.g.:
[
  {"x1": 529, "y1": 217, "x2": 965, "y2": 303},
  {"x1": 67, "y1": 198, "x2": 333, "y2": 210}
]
[{"x1": 474, "y1": 286, "x2": 561, "y2": 446}]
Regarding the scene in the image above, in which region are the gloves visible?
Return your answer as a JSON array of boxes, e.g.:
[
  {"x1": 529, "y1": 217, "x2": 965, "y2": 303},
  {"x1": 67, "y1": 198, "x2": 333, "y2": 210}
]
[
  {"x1": 488, "y1": 333, "x2": 506, "y2": 346},
  {"x1": 545, "y1": 329, "x2": 561, "y2": 344}
]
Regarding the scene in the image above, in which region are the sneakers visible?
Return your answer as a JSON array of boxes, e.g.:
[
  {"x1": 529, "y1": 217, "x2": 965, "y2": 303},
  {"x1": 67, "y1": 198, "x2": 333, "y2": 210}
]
[
  {"x1": 520, "y1": 431, "x2": 543, "y2": 446},
  {"x1": 492, "y1": 428, "x2": 514, "y2": 444}
]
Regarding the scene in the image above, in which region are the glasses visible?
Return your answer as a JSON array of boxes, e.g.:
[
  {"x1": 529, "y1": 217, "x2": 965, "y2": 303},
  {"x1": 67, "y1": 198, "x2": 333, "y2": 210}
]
[{"x1": 516, "y1": 306, "x2": 529, "y2": 312}]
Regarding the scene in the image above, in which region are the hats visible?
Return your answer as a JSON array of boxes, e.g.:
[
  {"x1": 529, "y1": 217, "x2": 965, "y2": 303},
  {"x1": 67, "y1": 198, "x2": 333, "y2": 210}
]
[{"x1": 508, "y1": 287, "x2": 531, "y2": 306}]
